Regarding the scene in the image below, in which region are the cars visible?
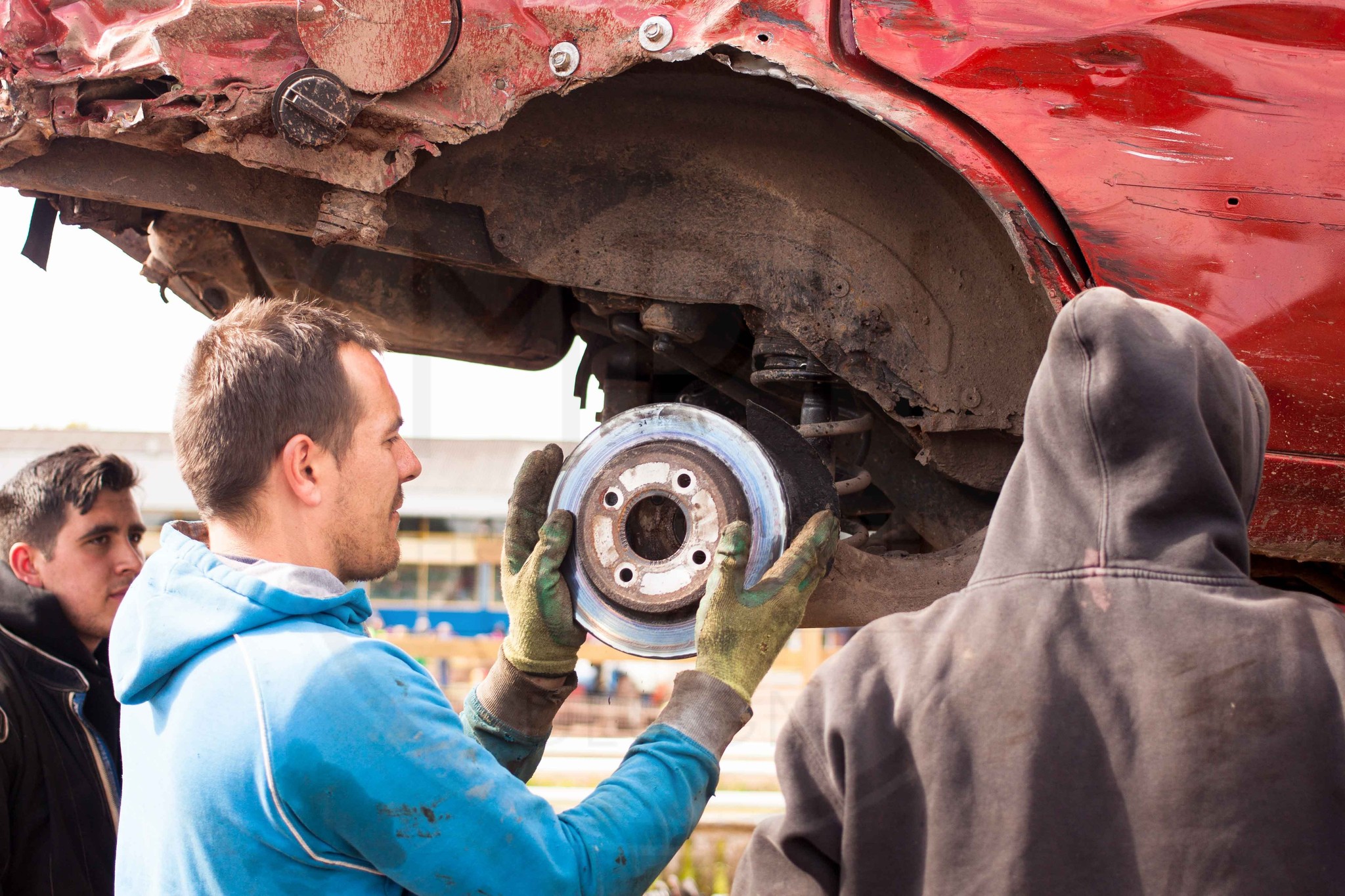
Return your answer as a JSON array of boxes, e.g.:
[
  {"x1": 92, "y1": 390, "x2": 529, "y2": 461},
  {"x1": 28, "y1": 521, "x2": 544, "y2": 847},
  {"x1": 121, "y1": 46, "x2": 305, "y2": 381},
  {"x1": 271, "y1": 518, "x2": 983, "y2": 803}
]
[{"x1": 0, "y1": 0, "x2": 1342, "y2": 656}]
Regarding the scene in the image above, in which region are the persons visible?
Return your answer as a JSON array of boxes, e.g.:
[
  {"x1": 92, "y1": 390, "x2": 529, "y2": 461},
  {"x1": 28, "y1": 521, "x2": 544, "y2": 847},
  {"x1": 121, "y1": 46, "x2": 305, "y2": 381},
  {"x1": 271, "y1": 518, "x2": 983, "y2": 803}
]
[
  {"x1": 0, "y1": 446, "x2": 144, "y2": 896},
  {"x1": 106, "y1": 292, "x2": 842, "y2": 896},
  {"x1": 729, "y1": 287, "x2": 1345, "y2": 896}
]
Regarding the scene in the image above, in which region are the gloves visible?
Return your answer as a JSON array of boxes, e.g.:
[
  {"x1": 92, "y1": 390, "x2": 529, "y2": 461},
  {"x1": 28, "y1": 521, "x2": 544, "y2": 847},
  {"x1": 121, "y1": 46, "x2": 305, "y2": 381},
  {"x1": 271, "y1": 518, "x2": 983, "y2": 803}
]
[
  {"x1": 501, "y1": 443, "x2": 587, "y2": 674},
  {"x1": 695, "y1": 509, "x2": 841, "y2": 705}
]
